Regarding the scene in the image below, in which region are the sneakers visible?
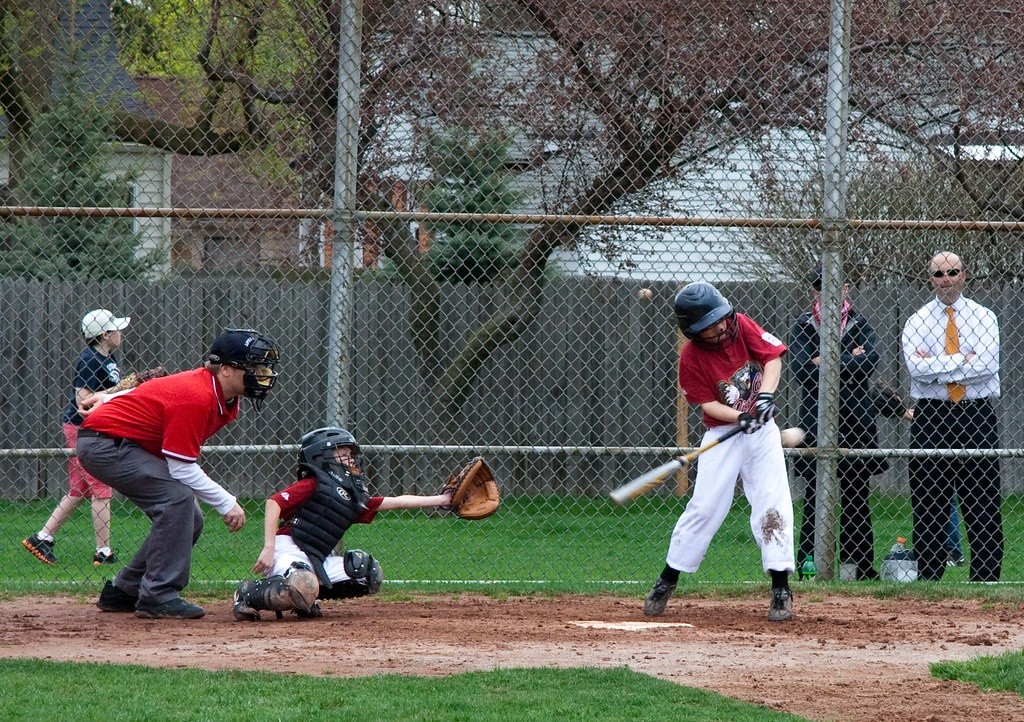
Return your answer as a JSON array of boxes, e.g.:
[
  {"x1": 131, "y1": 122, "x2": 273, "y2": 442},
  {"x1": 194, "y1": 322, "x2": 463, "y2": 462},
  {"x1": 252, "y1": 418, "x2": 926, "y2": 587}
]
[
  {"x1": 93, "y1": 549, "x2": 119, "y2": 566},
  {"x1": 644, "y1": 577, "x2": 682, "y2": 616},
  {"x1": 768, "y1": 587, "x2": 793, "y2": 621},
  {"x1": 22, "y1": 533, "x2": 59, "y2": 566},
  {"x1": 96, "y1": 580, "x2": 138, "y2": 612},
  {"x1": 135, "y1": 597, "x2": 205, "y2": 619}
]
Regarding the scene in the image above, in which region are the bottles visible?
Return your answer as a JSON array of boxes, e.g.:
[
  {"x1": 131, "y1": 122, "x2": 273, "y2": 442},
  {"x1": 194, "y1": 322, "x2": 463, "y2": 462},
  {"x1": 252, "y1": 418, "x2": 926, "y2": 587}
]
[
  {"x1": 890, "y1": 537, "x2": 906, "y2": 553},
  {"x1": 802, "y1": 555, "x2": 816, "y2": 580}
]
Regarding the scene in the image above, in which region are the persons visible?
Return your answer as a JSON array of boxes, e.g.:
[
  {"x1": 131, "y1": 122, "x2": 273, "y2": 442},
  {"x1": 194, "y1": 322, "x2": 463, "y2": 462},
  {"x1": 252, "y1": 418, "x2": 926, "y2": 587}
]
[
  {"x1": 947, "y1": 485, "x2": 965, "y2": 568},
  {"x1": 642, "y1": 281, "x2": 795, "y2": 622},
  {"x1": 790, "y1": 266, "x2": 890, "y2": 583},
  {"x1": 901, "y1": 250, "x2": 1003, "y2": 582},
  {"x1": 232, "y1": 426, "x2": 452, "y2": 620},
  {"x1": 75, "y1": 327, "x2": 279, "y2": 618},
  {"x1": 21, "y1": 309, "x2": 165, "y2": 566}
]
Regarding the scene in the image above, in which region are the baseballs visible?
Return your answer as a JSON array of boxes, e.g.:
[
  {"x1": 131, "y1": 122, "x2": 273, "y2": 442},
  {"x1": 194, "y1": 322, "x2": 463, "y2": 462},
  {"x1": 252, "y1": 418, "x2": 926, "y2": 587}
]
[{"x1": 782, "y1": 426, "x2": 800, "y2": 446}]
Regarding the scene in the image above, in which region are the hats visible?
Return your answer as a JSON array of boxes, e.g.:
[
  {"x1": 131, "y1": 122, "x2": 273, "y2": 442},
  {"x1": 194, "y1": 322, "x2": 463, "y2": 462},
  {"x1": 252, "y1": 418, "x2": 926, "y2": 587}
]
[
  {"x1": 812, "y1": 266, "x2": 850, "y2": 291},
  {"x1": 81, "y1": 309, "x2": 130, "y2": 338}
]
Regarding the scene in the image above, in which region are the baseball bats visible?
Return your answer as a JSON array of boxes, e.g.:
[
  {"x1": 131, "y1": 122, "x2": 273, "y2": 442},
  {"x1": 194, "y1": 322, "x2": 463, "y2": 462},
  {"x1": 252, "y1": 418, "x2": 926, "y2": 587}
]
[{"x1": 609, "y1": 421, "x2": 751, "y2": 504}]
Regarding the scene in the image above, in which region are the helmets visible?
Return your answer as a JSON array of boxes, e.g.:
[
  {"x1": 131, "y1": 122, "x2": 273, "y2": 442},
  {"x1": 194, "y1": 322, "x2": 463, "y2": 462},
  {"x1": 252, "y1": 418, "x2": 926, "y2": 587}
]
[
  {"x1": 674, "y1": 282, "x2": 738, "y2": 345},
  {"x1": 209, "y1": 328, "x2": 278, "y2": 365},
  {"x1": 297, "y1": 427, "x2": 371, "y2": 506}
]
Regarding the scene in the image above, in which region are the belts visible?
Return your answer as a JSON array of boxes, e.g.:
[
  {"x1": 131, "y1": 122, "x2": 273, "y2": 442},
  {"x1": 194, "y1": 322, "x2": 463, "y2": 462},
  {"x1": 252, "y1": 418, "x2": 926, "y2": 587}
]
[
  {"x1": 920, "y1": 398, "x2": 990, "y2": 408},
  {"x1": 77, "y1": 429, "x2": 108, "y2": 438}
]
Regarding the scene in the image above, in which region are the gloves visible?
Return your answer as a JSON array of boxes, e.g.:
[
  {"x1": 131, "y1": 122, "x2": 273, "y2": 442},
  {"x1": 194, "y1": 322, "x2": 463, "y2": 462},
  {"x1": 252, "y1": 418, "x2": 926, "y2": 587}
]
[
  {"x1": 738, "y1": 413, "x2": 762, "y2": 435},
  {"x1": 754, "y1": 392, "x2": 776, "y2": 424}
]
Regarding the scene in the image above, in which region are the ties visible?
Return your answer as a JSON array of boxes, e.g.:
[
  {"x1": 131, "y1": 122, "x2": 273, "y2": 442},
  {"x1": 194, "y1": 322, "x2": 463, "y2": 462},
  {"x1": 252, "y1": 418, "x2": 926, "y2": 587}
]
[{"x1": 943, "y1": 306, "x2": 967, "y2": 401}]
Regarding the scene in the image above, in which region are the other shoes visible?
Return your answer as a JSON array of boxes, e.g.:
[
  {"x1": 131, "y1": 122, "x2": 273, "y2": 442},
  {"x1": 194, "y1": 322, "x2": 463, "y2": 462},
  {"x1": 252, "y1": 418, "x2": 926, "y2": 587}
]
[
  {"x1": 293, "y1": 602, "x2": 323, "y2": 618},
  {"x1": 946, "y1": 553, "x2": 963, "y2": 567},
  {"x1": 233, "y1": 589, "x2": 261, "y2": 621}
]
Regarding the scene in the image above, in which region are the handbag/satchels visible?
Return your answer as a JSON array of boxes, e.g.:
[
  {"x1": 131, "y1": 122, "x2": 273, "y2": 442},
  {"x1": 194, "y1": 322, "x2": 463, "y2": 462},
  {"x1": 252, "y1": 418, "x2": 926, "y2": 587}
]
[{"x1": 881, "y1": 559, "x2": 918, "y2": 582}]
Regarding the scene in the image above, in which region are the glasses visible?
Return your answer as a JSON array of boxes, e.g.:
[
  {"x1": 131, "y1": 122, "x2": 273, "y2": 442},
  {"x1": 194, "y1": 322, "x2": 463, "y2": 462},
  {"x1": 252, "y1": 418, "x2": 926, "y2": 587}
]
[{"x1": 931, "y1": 268, "x2": 963, "y2": 279}]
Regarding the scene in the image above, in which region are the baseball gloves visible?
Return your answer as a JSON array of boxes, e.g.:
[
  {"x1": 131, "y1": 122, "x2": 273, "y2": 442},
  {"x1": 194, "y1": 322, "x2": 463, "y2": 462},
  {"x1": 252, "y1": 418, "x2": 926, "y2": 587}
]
[{"x1": 440, "y1": 455, "x2": 501, "y2": 520}]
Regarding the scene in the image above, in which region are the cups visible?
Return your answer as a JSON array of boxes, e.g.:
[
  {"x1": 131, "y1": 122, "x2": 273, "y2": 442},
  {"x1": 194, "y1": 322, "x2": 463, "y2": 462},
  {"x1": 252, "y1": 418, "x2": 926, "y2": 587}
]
[{"x1": 839, "y1": 564, "x2": 856, "y2": 581}]
